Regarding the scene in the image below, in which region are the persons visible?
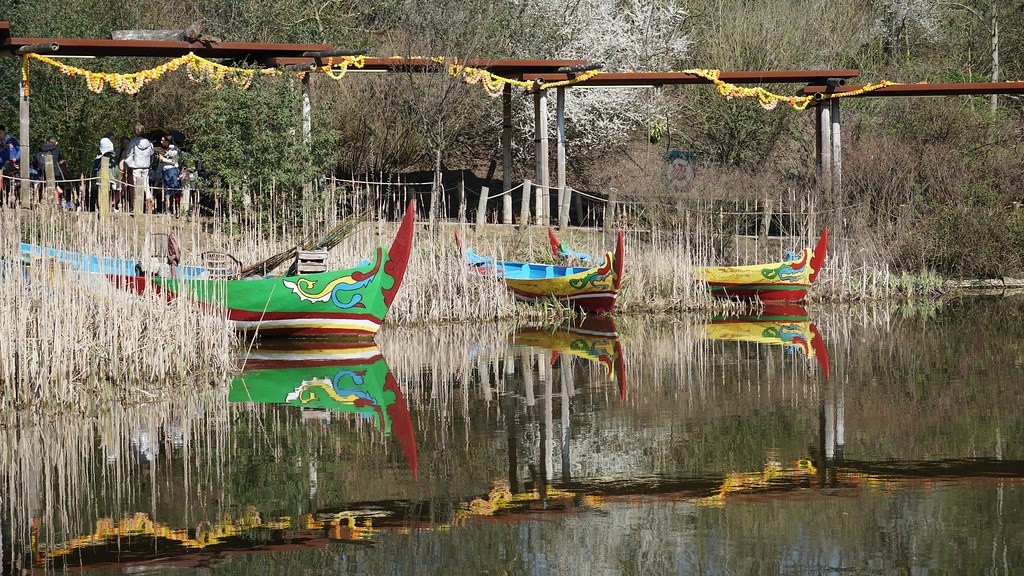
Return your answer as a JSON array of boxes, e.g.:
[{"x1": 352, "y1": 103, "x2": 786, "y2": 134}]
[
  {"x1": 155, "y1": 135, "x2": 180, "y2": 215},
  {"x1": 91, "y1": 123, "x2": 155, "y2": 214},
  {"x1": 0, "y1": 124, "x2": 20, "y2": 208},
  {"x1": 34, "y1": 136, "x2": 64, "y2": 203}
]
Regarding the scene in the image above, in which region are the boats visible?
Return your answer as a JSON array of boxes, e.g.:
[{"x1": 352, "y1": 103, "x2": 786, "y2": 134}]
[
  {"x1": 0, "y1": 195, "x2": 416, "y2": 337},
  {"x1": 219, "y1": 330, "x2": 420, "y2": 484},
  {"x1": 451, "y1": 228, "x2": 626, "y2": 315},
  {"x1": 683, "y1": 225, "x2": 830, "y2": 304},
  {"x1": 511, "y1": 309, "x2": 630, "y2": 404},
  {"x1": 546, "y1": 226, "x2": 605, "y2": 267},
  {"x1": 693, "y1": 305, "x2": 832, "y2": 379}
]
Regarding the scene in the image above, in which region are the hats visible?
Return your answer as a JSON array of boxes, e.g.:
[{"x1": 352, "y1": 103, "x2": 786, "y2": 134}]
[{"x1": 95, "y1": 138, "x2": 115, "y2": 159}]
